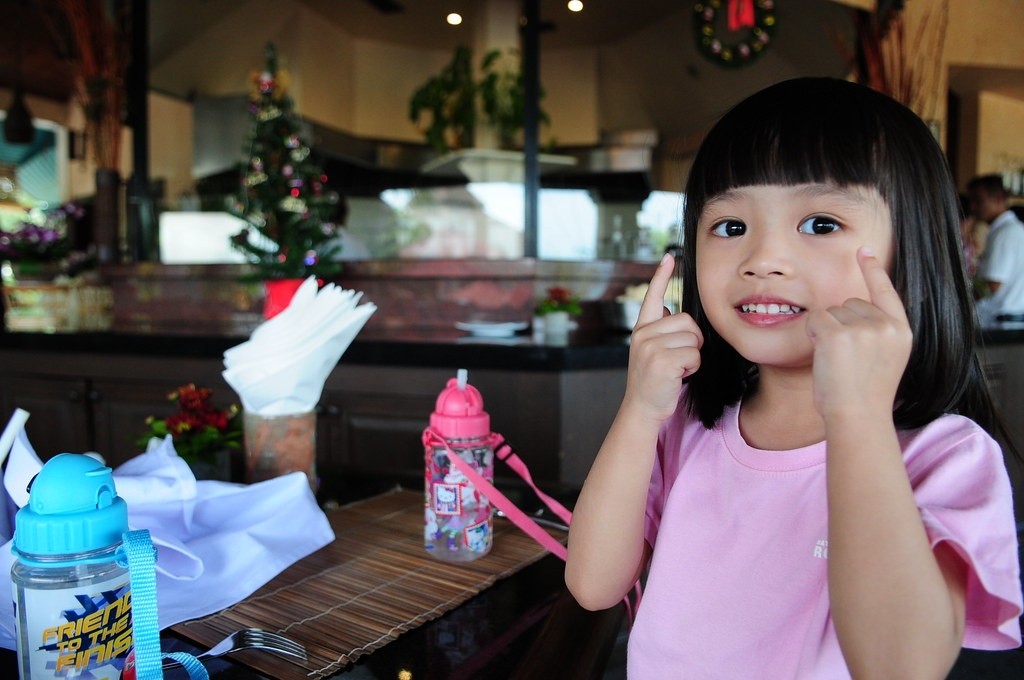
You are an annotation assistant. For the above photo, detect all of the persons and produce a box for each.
[956,191,980,273]
[963,170,1024,346]
[561,74,1023,680]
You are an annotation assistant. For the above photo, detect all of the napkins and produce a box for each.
[222,273,377,416]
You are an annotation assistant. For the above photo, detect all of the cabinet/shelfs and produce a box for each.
[0,370,209,470]
[224,395,503,479]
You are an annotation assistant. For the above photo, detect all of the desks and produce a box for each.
[0,478,626,680]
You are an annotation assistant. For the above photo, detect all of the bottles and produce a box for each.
[9,454,139,680]
[423,370,495,561]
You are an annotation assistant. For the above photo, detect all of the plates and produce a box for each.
[455,322,529,336]
